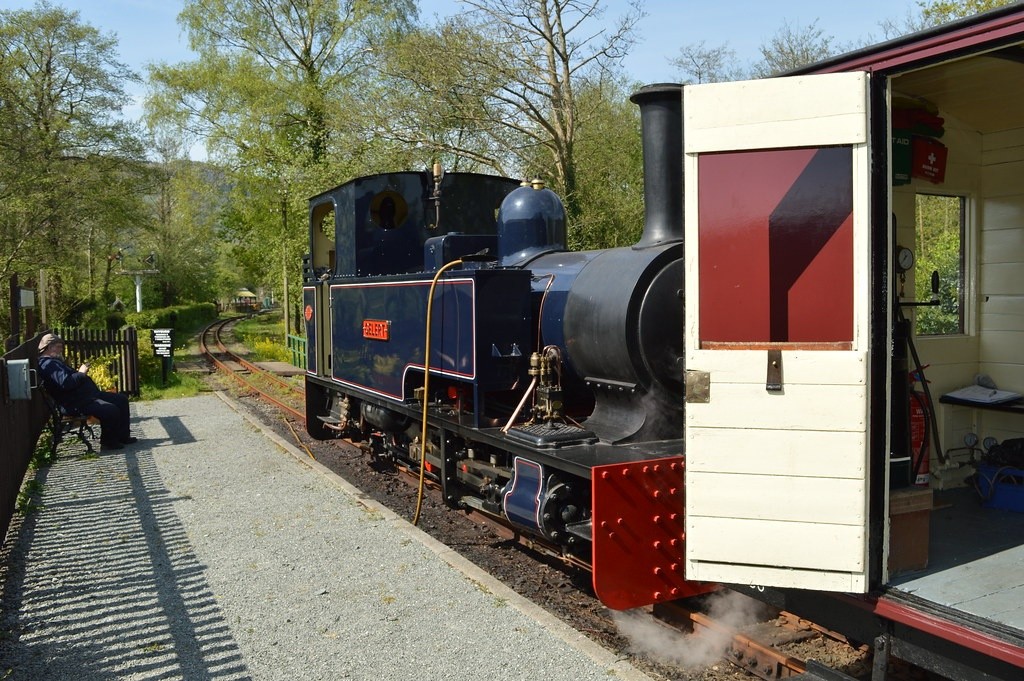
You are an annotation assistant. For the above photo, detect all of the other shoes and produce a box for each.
[120,435,138,444]
[101,444,125,450]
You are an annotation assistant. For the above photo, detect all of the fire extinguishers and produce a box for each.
[909,364,931,487]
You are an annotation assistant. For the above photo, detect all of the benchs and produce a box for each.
[937,385,1024,414]
[42,380,99,453]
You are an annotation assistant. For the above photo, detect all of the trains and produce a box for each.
[302,1,1023,681]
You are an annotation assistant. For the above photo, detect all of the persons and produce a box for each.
[36,333,136,450]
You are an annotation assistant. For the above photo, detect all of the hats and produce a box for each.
[38,333,59,354]
